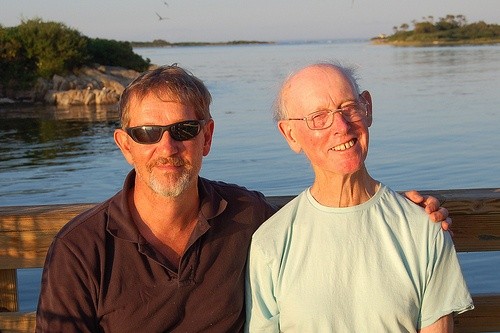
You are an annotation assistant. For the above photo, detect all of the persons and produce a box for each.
[243,60,475,333]
[34,65,453,333]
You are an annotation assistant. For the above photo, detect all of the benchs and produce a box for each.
[2,187,500,332]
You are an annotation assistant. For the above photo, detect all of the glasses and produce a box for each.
[126,116,206,144]
[282,94,370,130]
